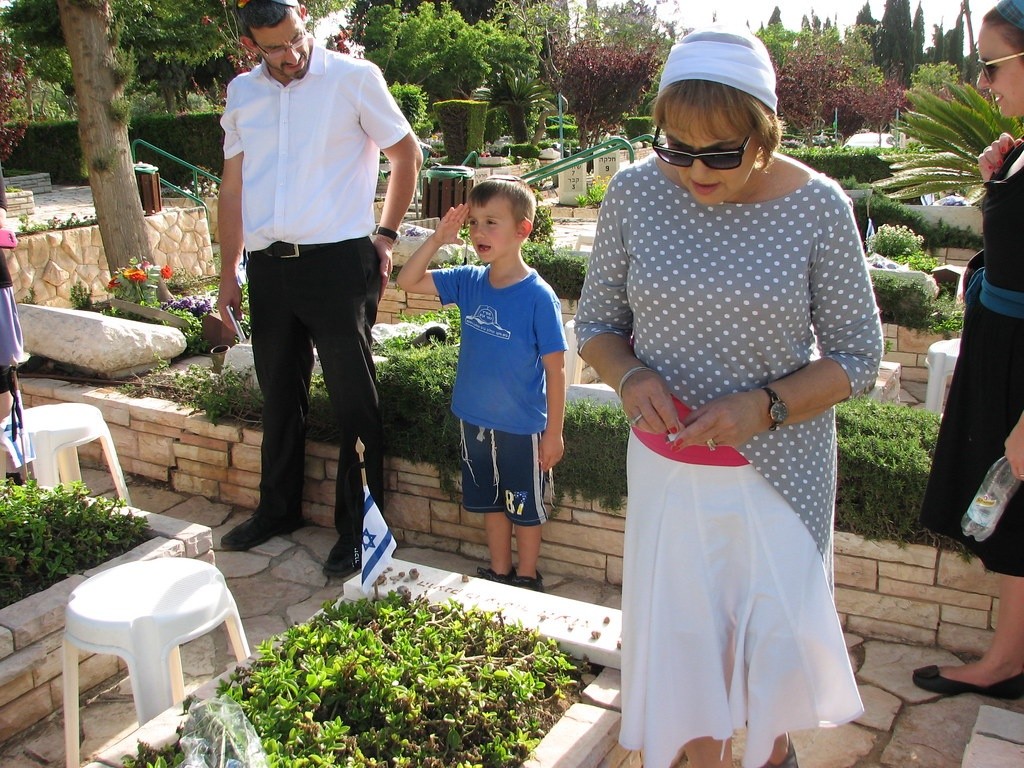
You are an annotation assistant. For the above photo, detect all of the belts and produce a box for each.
[261,242,329,259]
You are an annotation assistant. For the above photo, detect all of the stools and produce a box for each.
[0,401,132,507]
[923,337,961,414]
[61,557,251,768]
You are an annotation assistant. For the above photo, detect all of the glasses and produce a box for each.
[975,50,1024,85]
[254,27,308,53]
[650,121,756,171]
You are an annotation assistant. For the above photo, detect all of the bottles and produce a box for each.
[960,456,1021,543]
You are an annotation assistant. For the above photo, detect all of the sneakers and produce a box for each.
[477,564,545,592]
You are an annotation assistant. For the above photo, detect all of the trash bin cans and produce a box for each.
[132,161,162,216]
[421,166,476,219]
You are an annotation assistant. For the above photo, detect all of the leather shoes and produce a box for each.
[324,535,361,577]
[222,513,308,552]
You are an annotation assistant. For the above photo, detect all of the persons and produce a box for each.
[396,174,569,593]
[573,22,883,768]
[217,0,424,578]
[912,0,1023,701]
[0,164,28,485]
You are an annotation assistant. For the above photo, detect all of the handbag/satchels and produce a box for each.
[963,135,1024,298]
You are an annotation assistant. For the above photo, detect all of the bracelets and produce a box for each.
[376,227,398,241]
[618,366,657,399]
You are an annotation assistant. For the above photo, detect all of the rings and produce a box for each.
[707,438,717,453]
[631,413,643,427]
[1019,474,1024,477]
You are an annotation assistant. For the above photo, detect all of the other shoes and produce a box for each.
[765,734,798,768]
[5,472,24,486]
[912,665,1024,700]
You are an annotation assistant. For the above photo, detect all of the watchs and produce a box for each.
[760,386,790,433]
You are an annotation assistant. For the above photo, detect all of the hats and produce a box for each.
[658,23,778,113]
[235,0,297,18]
